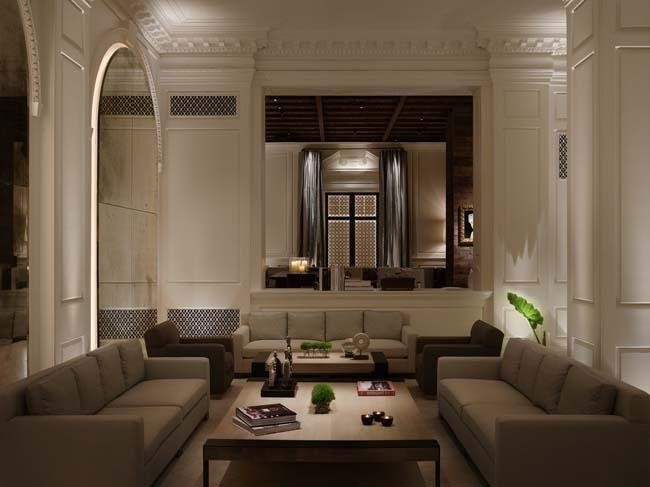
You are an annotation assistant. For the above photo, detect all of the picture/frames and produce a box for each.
[458,206,473,247]
[289,256,310,274]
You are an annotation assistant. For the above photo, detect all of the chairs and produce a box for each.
[415,319,504,400]
[424,267,449,289]
[144,320,235,399]
[380,277,416,287]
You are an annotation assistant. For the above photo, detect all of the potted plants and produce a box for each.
[312,383,335,413]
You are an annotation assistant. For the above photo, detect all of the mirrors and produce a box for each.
[1,0,30,347]
[96,46,159,358]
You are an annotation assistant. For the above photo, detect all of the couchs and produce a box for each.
[1,306,29,346]
[437,337,650,486]
[232,309,419,379]
[0,339,211,486]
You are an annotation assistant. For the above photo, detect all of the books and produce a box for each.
[236,403,296,427]
[357,380,395,396]
[232,416,301,436]
[317,264,375,290]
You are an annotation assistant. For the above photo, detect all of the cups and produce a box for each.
[361,414,373,424]
[381,415,393,426]
[373,411,385,421]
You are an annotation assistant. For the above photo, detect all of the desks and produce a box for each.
[266,268,317,288]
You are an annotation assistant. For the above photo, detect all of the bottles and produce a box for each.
[268,345,295,386]
